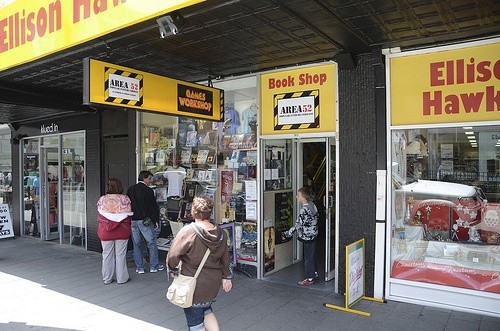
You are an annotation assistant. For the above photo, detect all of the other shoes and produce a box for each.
[315,275,318,280]
[298,279,315,285]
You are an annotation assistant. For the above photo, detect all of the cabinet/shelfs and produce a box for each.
[141,126,259,262]
[45,163,85,225]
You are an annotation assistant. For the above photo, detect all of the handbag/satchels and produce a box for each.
[166,274,197,308]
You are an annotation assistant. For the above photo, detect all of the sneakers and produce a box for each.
[150,264,164,272]
[136,269,144,273]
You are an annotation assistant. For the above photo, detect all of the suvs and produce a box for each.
[391,162,487,232]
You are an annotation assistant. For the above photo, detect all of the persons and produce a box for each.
[282,187,319,286]
[97,178,132,285]
[167,194,233,331]
[126,171,165,274]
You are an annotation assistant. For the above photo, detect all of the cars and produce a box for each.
[150,170,247,220]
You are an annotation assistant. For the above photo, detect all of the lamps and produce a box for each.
[156,13,189,39]
